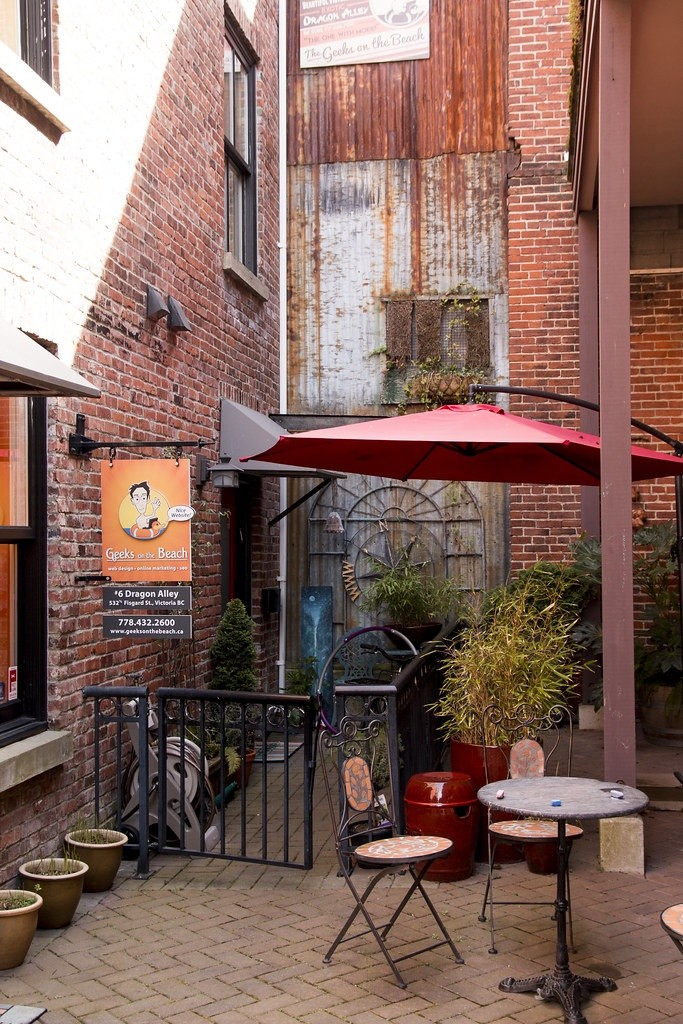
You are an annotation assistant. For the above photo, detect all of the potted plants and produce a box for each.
[18,845,90,928]
[416,280,482,395]
[630,520,683,742]
[206,598,262,788]
[64,818,129,893]
[0,883,43,971]
[426,564,599,866]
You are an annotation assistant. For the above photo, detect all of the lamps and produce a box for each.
[194,453,243,487]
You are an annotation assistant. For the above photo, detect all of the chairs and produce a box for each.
[659,901,682,955]
[320,716,465,990]
[476,705,579,952]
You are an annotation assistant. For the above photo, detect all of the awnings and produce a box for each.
[240,402,683,487]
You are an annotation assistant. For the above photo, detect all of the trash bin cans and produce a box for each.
[403,770,480,884]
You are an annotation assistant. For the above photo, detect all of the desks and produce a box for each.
[475,777,649,1024]
